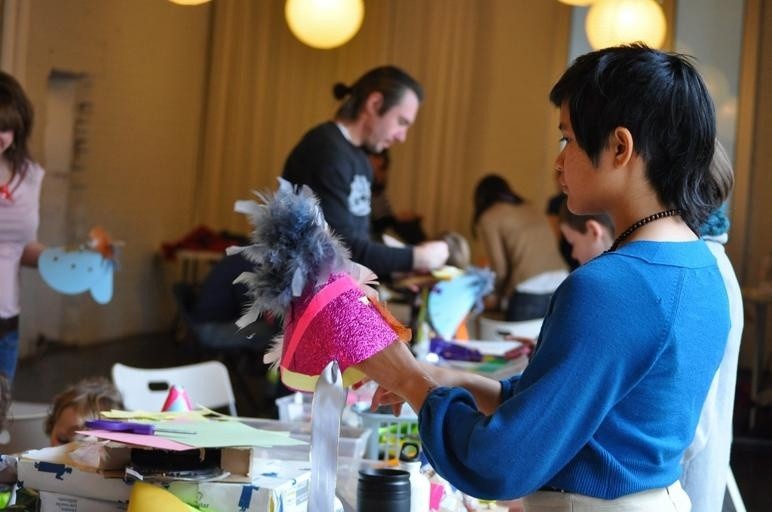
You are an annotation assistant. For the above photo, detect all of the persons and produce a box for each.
[0,69,52,456]
[467,174,570,323]
[190,255,281,411]
[44,378,126,445]
[677,133,747,511]
[546,180,618,273]
[280,64,449,274]
[362,143,432,246]
[348,45,746,511]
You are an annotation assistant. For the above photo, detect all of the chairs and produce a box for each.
[111,360,239,419]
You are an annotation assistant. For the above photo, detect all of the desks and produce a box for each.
[175,246,471,355]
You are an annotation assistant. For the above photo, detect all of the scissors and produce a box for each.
[84,418,199,436]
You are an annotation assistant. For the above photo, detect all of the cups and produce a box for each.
[357,468,411,512]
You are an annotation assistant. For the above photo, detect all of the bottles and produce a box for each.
[401,461,430,511]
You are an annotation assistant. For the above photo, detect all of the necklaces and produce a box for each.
[609,207,684,250]
